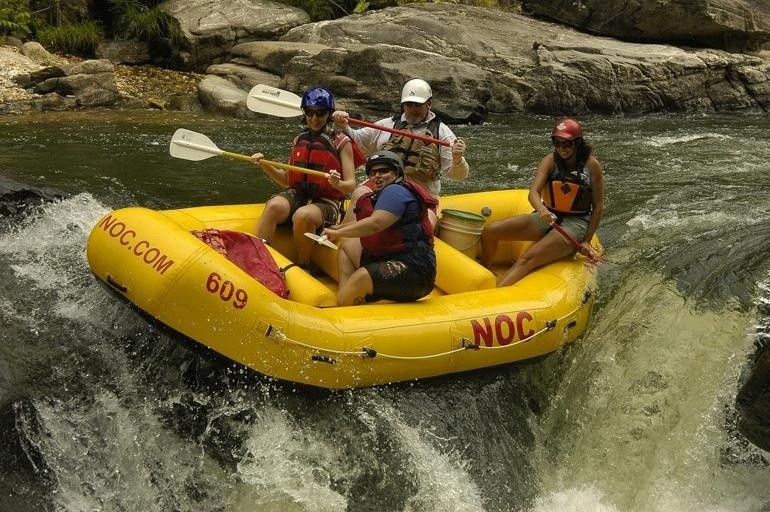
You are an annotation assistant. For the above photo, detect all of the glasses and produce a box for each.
[554,141,573,148]
[304,109,327,117]
[406,102,423,107]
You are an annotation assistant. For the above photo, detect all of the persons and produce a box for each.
[320,149,437,307]
[252,88,356,278]
[477,118,604,288]
[333,78,470,238]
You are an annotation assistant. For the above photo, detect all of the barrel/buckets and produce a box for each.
[439,209,487,259]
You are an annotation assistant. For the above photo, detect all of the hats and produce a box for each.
[400,78,432,103]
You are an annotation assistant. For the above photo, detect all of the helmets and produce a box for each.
[365,149,405,179]
[300,86,335,111]
[549,119,583,141]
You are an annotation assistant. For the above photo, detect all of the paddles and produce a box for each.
[246,84,454,149]
[170,127,330,181]
[305,231,338,250]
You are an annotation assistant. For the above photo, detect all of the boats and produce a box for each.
[86,189,604,390]
